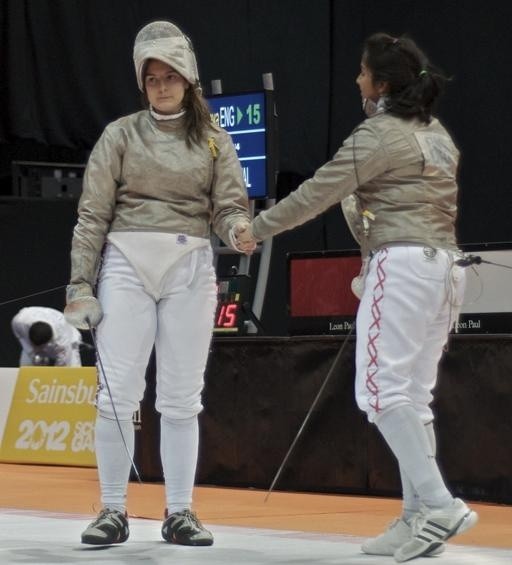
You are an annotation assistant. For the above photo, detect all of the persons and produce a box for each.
[11,305,83,368]
[63,21,257,544]
[238,36,477,564]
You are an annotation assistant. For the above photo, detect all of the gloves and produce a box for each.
[64,282,102,331]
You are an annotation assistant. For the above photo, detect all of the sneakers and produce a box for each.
[393,497,479,563]
[361,517,445,557]
[161,508,213,547]
[81,507,131,545]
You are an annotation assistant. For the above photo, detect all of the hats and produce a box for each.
[133,20,199,91]
